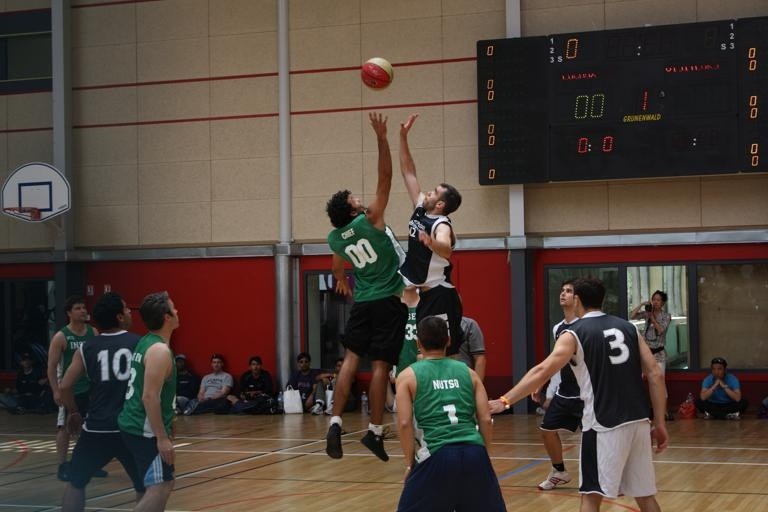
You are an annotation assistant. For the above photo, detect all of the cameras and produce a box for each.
[645,304,652,312]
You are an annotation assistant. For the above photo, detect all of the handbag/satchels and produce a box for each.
[284,386,303,414]
[325,390,334,409]
[679,400,696,419]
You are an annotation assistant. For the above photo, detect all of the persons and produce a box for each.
[396,315,508,512]
[2,350,55,417]
[324,112,410,462]
[488,276,671,511]
[58,291,144,510]
[47,297,110,480]
[532,279,588,492]
[700,358,749,419]
[395,114,466,357]
[173,352,357,416]
[391,284,421,418]
[630,291,671,368]
[117,291,181,511]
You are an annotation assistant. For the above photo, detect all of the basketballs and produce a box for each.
[362,59,393,89]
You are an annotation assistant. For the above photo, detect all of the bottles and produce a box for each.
[277,391,283,412]
[361,390,369,415]
[686,392,693,402]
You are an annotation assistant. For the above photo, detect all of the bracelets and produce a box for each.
[501,394,510,410]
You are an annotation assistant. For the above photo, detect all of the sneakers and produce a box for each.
[94,469,108,478]
[57,462,72,480]
[326,423,343,458]
[311,404,323,414]
[360,430,389,462]
[704,411,713,419]
[727,411,741,421]
[324,406,333,415]
[538,466,572,490]
